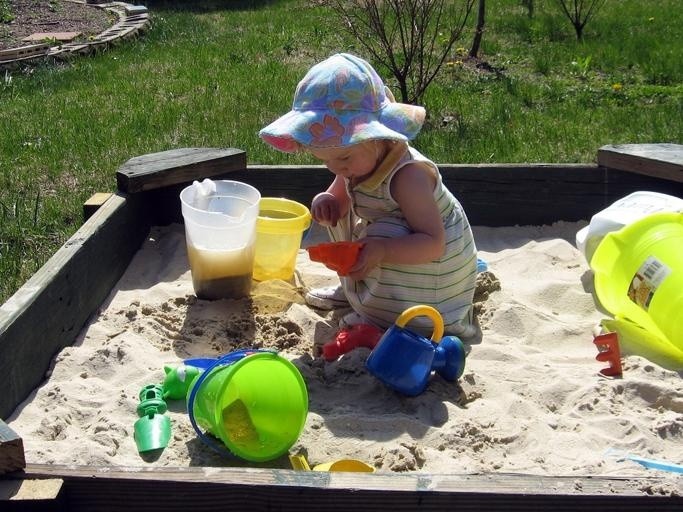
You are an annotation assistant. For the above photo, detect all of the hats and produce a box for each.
[257,53,426,155]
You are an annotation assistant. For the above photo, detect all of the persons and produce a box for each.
[260,54,476,334]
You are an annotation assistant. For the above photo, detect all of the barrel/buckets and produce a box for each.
[589,211,683,354]
[185,350,309,460]
[179,176,261,300]
[254,198,311,284]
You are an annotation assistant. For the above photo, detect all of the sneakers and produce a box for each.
[339,311,368,330]
[306,282,352,309]
